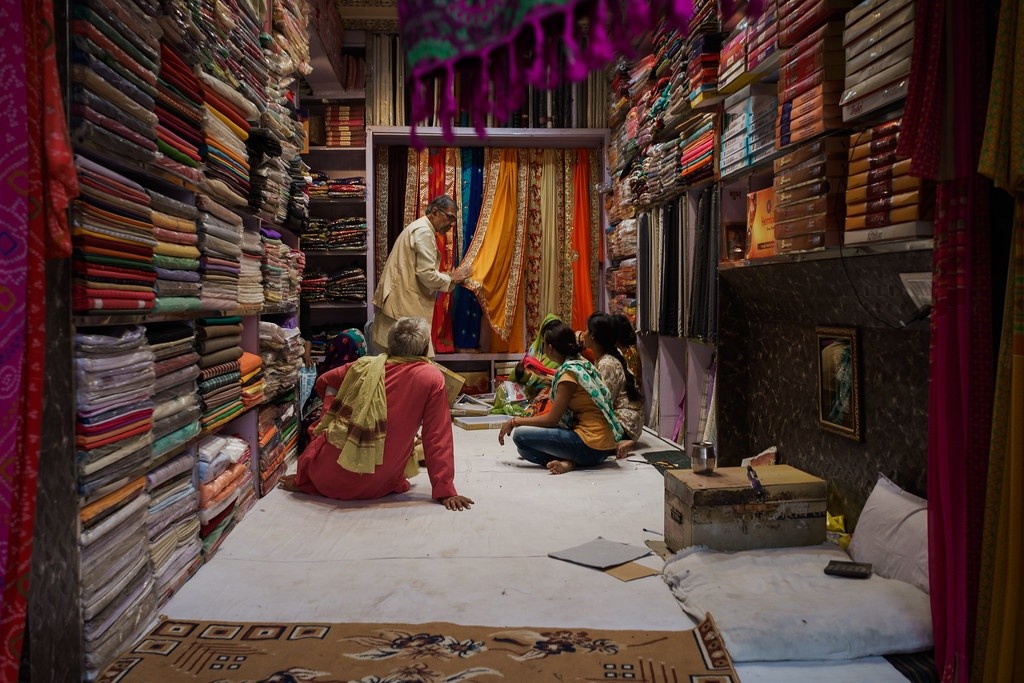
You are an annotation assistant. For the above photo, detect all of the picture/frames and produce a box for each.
[814,326,859,443]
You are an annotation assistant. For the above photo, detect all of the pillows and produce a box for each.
[661,543,932,661]
[845,471,929,596]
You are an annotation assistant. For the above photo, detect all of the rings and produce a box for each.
[450,500,453,503]
[499,435,502,437]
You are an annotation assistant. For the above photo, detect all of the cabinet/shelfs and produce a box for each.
[26,1,376,683]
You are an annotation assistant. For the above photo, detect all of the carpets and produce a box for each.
[89,611,746,683]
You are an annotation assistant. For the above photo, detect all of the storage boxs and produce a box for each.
[718,1,934,260]
[664,464,828,553]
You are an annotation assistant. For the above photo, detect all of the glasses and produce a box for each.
[438,209,457,222]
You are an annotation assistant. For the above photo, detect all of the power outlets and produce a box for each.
[899,272,933,318]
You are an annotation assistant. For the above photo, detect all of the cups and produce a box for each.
[692,442,715,473]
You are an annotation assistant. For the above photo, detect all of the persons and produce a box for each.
[498,311,645,475]
[278,316,474,511]
[299,296,316,368]
[371,195,474,356]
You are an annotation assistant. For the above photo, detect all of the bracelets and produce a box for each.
[509,418,519,428]
